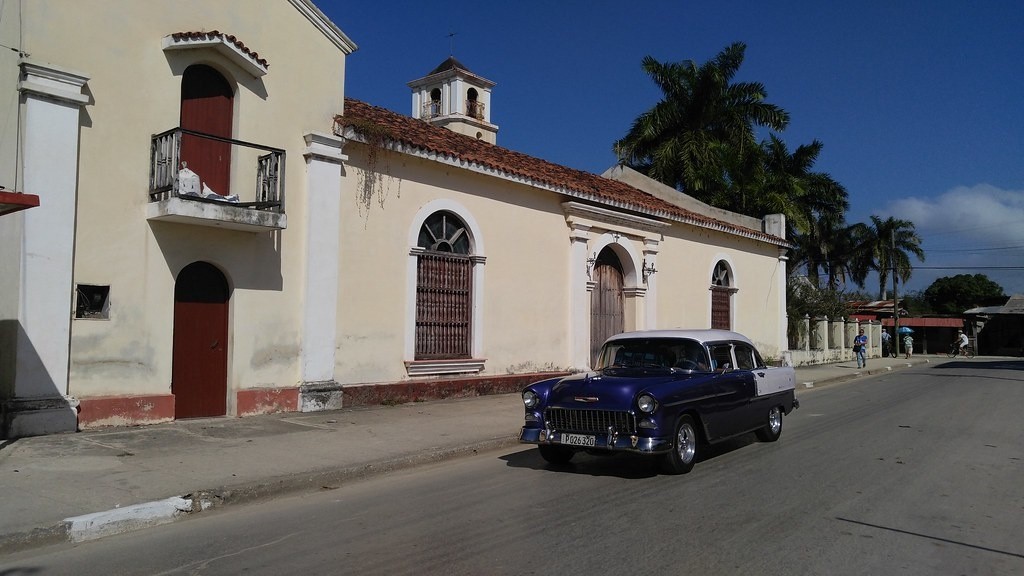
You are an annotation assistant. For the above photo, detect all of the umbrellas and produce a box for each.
[896,327,915,333]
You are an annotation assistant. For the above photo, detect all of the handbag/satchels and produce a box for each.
[882,334,888,342]
[853,345,861,352]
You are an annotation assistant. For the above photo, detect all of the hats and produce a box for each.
[958,330,963,332]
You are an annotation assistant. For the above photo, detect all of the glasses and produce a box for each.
[860,332,864,333]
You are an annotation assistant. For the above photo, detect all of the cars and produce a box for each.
[520,328,802,473]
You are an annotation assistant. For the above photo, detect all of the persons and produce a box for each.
[882,329,891,342]
[903,333,914,359]
[854,329,867,369]
[955,330,969,356]
[674,345,717,372]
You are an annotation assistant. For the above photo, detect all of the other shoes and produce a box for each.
[863,363,866,368]
[959,349,962,351]
[905,358,907,359]
[857,367,861,369]
[965,352,968,355]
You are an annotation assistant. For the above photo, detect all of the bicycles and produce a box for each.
[882,341,897,358]
[947,342,976,359]
